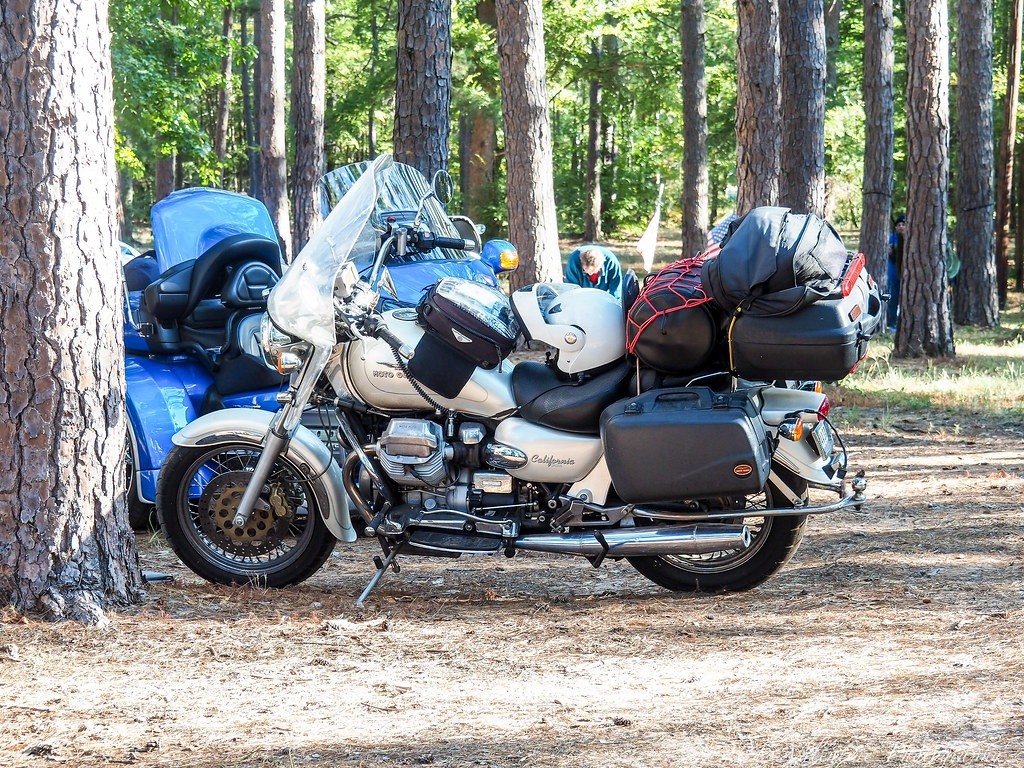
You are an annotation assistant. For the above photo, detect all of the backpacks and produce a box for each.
[414,275,528,371]
[945,240,960,279]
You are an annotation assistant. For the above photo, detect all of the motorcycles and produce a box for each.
[156,152,891,607]
[119,161,519,532]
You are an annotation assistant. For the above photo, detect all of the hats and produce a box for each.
[894,217,905,226]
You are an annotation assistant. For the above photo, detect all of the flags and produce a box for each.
[708,214,737,259]
[636,192,661,274]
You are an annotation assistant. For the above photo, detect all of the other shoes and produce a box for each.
[886,324,897,332]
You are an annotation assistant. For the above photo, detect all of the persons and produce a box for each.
[886,216,907,333]
[565,245,623,303]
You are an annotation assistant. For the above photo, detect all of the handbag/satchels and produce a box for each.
[701,205,847,320]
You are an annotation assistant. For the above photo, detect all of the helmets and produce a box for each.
[510,277,626,381]
[480,239,519,276]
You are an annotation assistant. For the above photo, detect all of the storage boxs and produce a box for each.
[602,386,776,507]
[730,264,889,389]
